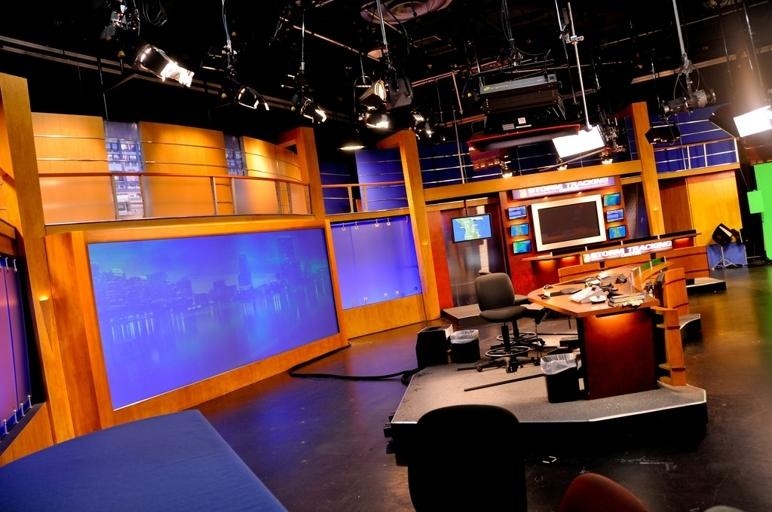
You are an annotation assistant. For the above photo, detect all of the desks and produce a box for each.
[521,229,711,284]
[526,256,690,400]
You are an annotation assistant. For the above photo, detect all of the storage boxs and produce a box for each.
[417,324,453,369]
[450,329,481,363]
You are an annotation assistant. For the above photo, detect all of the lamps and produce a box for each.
[545,0,627,171]
[217,0,269,111]
[452,73,472,141]
[645,55,681,144]
[280,0,328,123]
[733,0,772,138]
[662,0,717,113]
[502,155,513,177]
[118,0,195,89]
[338,0,446,149]
[708,0,740,139]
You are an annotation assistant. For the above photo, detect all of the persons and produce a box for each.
[559,472,649,512]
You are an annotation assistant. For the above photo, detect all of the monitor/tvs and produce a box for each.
[606,208,624,223]
[451,213,493,244]
[603,192,621,208]
[530,194,607,252]
[608,224,626,241]
[507,205,527,221]
[510,223,529,238]
[512,239,532,255]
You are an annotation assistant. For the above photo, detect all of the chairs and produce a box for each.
[474,270,545,373]
[408,405,527,512]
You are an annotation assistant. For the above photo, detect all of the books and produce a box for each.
[608,291,646,307]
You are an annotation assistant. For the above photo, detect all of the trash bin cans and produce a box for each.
[541,353,579,403]
[450,329,480,370]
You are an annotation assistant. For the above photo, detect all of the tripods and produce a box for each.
[712,246,738,270]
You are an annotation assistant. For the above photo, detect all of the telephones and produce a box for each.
[570,285,602,304]
